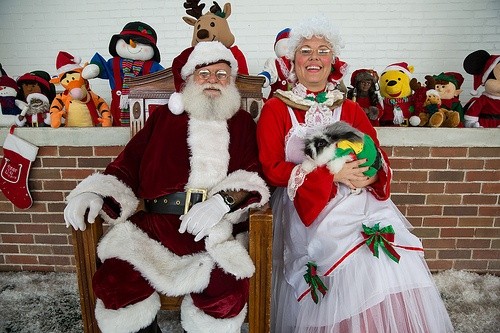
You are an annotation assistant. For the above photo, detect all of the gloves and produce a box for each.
[63,192,104,231]
[178,194,230,242]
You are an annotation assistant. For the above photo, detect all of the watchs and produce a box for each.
[220,193,235,207]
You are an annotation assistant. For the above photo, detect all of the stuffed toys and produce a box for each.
[81,21,166,127]
[377,62,464,128]
[258,28,298,101]
[49,51,113,128]
[182,0,248,75]
[0,63,56,127]
[463,50,500,128]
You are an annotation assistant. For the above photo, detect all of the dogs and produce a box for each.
[303,121,383,176]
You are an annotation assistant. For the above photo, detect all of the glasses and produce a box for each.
[189,69,233,81]
[296,45,333,56]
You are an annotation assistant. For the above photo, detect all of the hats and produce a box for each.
[167,41,238,115]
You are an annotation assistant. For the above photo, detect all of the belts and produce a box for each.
[135,189,208,215]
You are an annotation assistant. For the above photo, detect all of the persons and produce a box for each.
[64,41,271,333]
[257,22,454,333]
[347,69,384,127]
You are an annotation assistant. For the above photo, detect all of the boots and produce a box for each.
[134,314,162,333]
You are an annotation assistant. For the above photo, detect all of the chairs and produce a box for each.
[72,66,273,333]
